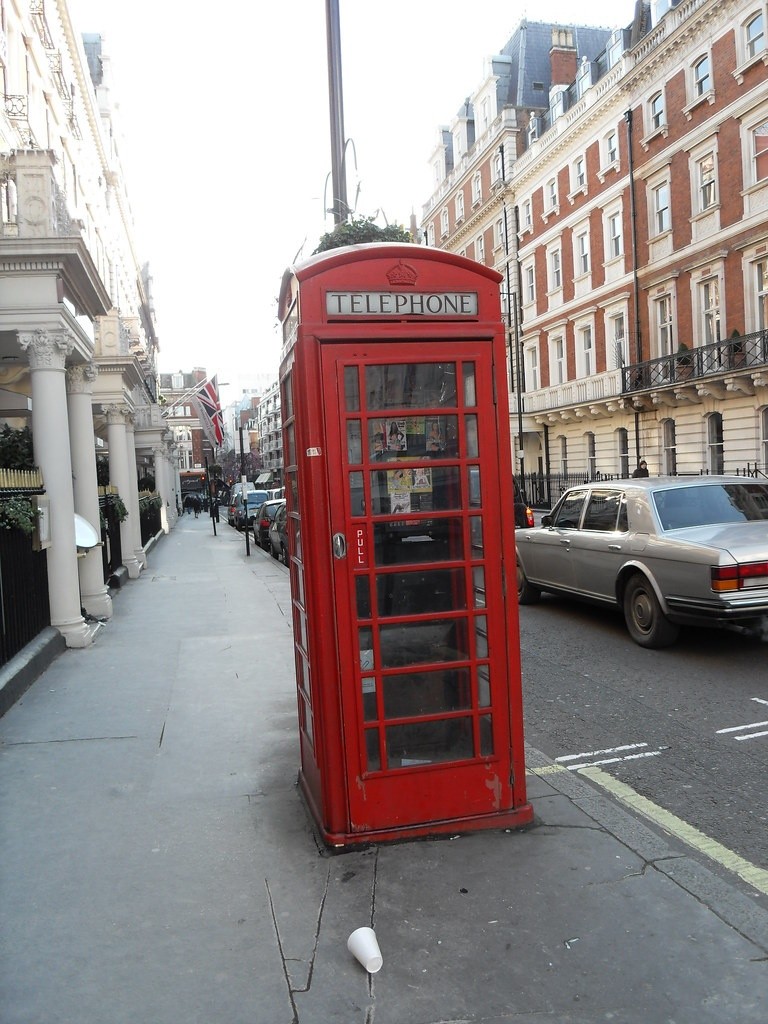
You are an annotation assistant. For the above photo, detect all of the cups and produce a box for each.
[347,927,383,973]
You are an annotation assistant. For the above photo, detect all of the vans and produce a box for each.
[230,482,255,506]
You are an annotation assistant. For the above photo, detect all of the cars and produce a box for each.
[515,475,768,651]
[249,498,287,551]
[224,494,237,526]
[425,470,535,545]
[264,502,287,567]
[232,490,269,531]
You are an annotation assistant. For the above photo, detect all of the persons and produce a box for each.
[632,461,649,478]
[388,422,405,450]
[184,494,203,518]
[429,423,444,451]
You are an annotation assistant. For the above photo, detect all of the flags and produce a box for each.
[189,374,224,449]
